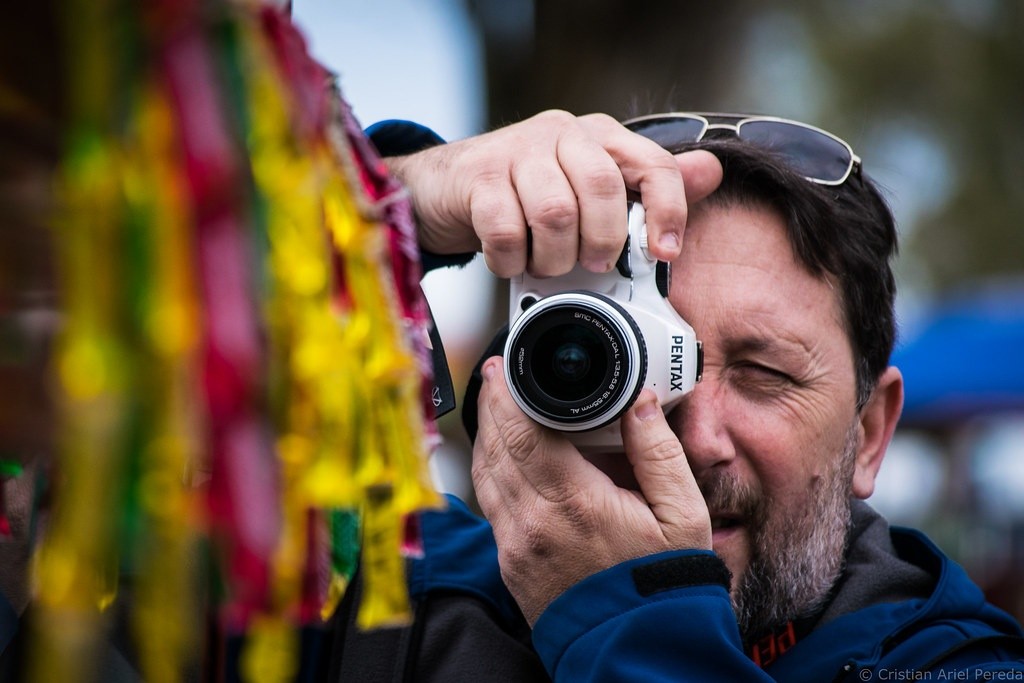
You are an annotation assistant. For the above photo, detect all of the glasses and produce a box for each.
[585,109,866,200]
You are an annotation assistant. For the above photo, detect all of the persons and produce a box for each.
[24,110,1024,683]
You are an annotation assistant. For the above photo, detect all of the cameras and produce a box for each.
[502,189,704,452]
